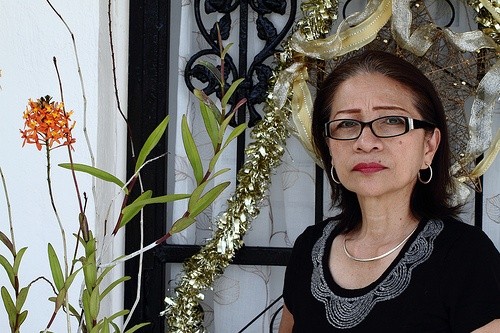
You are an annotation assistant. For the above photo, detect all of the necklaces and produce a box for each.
[341,222,419,263]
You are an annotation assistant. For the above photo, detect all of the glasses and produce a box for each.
[322,114,435,140]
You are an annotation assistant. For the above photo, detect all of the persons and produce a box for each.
[276,48,500,332]
